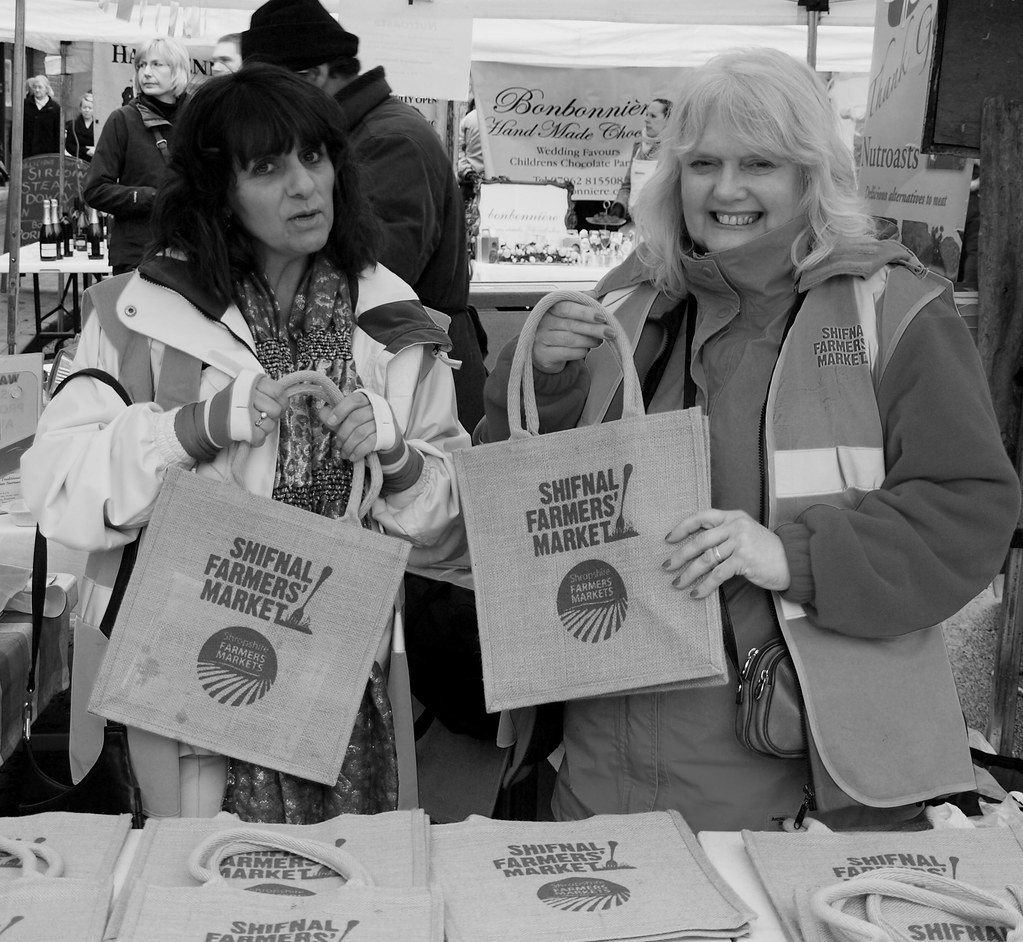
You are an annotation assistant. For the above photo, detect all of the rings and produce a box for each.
[713,546,723,563]
[255,412,267,426]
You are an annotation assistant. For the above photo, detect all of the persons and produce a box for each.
[457,109,484,206]
[221,0,488,446]
[9,74,60,159]
[84,32,195,276]
[610,98,674,223]
[472,46,1021,831]
[210,32,242,76]
[65,93,94,162]
[20,59,480,822]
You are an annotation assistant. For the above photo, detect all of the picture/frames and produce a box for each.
[468,174,576,261]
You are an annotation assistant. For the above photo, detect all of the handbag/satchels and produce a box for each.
[87,370,413,787]
[0,800,1023,942]
[452,290,729,713]
[737,638,812,758]
[0,687,143,829]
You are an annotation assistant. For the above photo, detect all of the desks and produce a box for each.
[468,259,609,310]
[0,611,70,768]
[697,831,789,942]
[0,239,112,358]
[0,809,758,942]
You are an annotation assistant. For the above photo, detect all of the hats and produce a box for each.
[240,0,359,73]
[28,74,54,98]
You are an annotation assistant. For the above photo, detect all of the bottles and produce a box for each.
[59,212,74,257]
[50,199,64,259]
[71,197,80,248]
[40,200,57,260]
[107,213,114,248]
[86,207,104,259]
[75,202,88,251]
